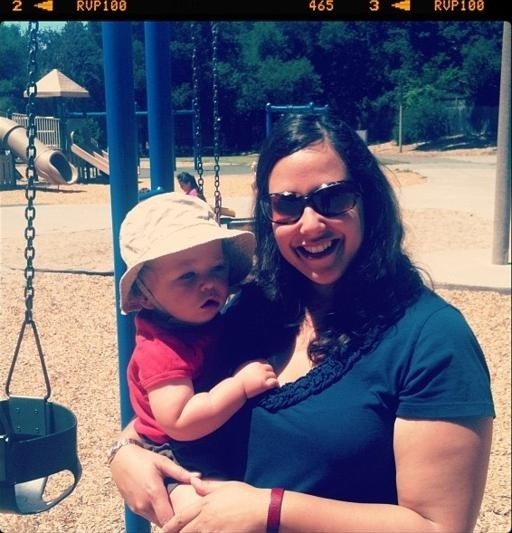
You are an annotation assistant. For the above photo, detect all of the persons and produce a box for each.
[117,191,279,517]
[105,107,496,533]
[177,171,202,197]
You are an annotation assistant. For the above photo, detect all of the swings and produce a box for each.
[189,20,221,226]
[0,20,82,514]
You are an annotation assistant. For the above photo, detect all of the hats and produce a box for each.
[119,193,256,316]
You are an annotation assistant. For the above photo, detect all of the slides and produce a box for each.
[71,144,109,176]
[0,116,79,185]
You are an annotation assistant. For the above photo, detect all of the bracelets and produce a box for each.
[265,487,285,533]
[104,438,144,468]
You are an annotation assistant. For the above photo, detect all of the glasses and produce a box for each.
[260,180,360,224]
